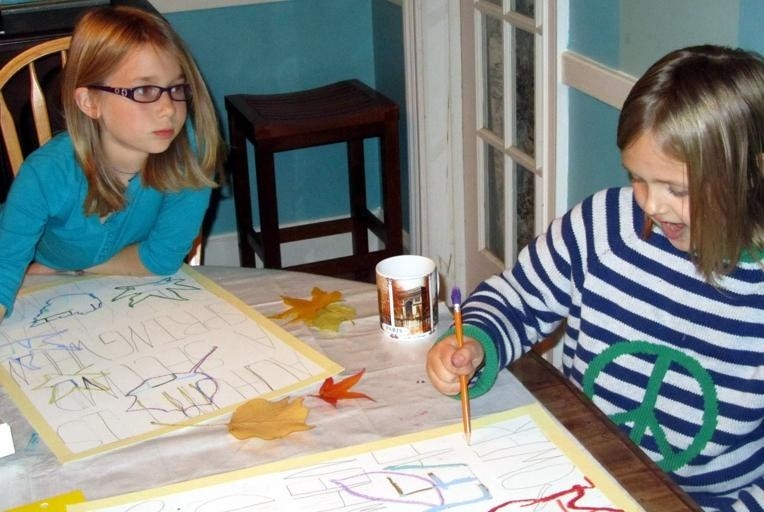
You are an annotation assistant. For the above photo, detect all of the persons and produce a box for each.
[0,6,222,331]
[425,45,764,512]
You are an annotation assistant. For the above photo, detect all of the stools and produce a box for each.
[225,80,402,280]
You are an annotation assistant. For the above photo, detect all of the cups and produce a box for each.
[376,253,441,340]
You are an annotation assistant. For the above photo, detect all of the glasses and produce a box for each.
[87,84,193,103]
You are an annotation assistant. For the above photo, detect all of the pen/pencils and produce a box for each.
[451,289,472,447]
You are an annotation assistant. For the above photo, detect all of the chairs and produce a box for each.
[0,36,202,266]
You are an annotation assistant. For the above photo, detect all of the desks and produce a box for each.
[0,266,703,512]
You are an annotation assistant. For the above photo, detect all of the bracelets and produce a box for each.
[72,269,85,276]
